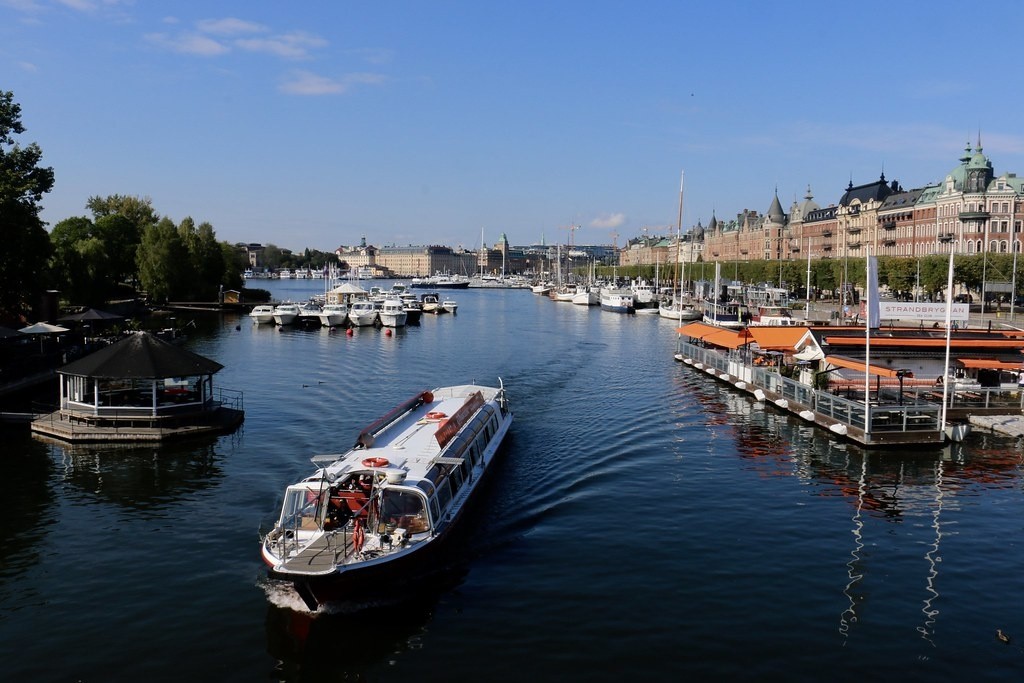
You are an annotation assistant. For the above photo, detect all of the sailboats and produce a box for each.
[466,168,815,331]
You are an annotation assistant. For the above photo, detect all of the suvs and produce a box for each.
[956,294,973,303]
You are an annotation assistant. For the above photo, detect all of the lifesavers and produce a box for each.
[353,520,364,552]
[362,457,389,467]
[426,412,446,418]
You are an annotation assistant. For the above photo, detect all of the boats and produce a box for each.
[442,300,458,313]
[377,299,408,327]
[249,305,275,325]
[298,298,322,327]
[935,418,973,444]
[421,292,440,314]
[403,306,422,325]
[243,265,374,280]
[319,303,348,327]
[369,283,421,309]
[272,303,300,326]
[259,384,514,580]
[347,302,379,328]
[410,264,471,289]
